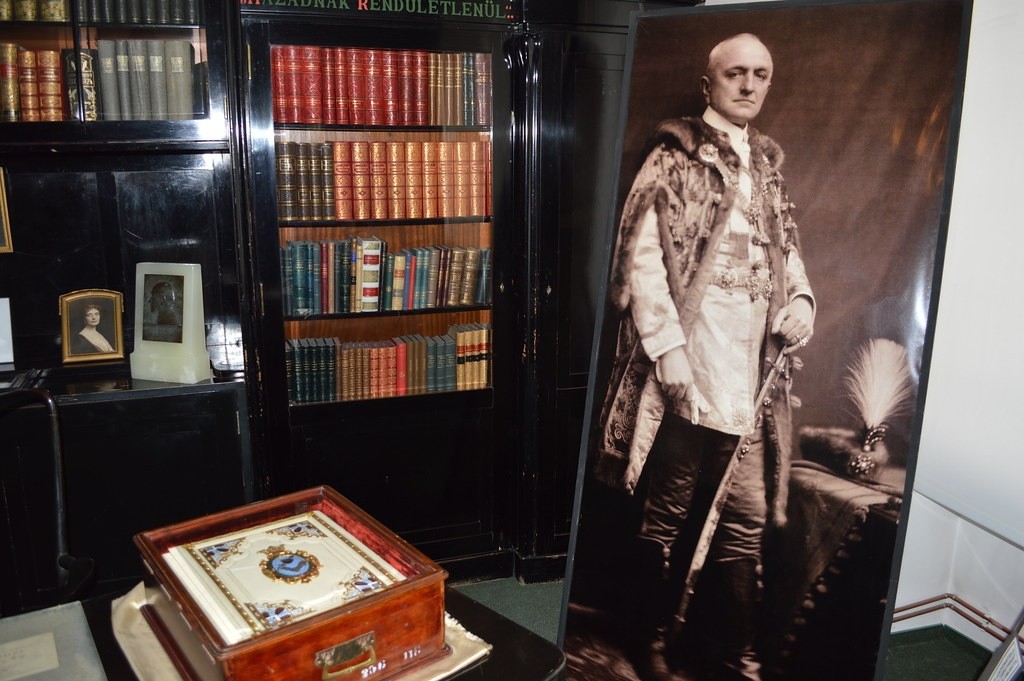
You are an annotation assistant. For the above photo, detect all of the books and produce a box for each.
[0,7,493,407]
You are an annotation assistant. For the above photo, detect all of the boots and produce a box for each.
[627,534,676,681]
[717,556,762,681]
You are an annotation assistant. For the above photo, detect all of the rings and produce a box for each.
[800,338,808,346]
[795,334,802,341]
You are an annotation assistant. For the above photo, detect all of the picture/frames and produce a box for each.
[0,167,13,253]
[557,0,975,681]
[59,289,124,368]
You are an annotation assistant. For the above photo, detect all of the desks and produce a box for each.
[81,584,568,681]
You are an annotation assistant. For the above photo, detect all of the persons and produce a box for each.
[72,306,113,355]
[592,33,817,681]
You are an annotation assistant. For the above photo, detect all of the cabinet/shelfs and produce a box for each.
[0,2,704,611]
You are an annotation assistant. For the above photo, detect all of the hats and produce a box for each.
[801,339,914,485]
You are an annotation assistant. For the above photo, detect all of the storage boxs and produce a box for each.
[134,485,453,681]
